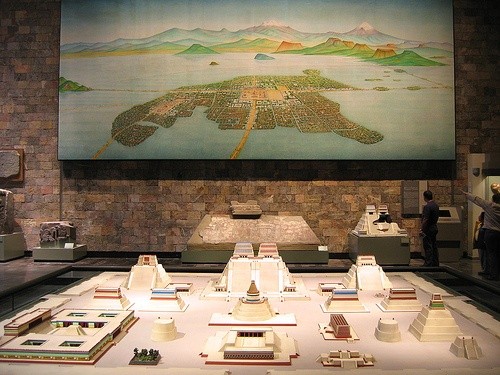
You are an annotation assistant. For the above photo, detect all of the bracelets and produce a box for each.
[420,229,422,230]
[463,192,466,196]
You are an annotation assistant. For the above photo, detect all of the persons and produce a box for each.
[456,183,500,280]
[419,190,440,267]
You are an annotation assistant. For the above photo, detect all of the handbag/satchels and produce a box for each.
[472,228,487,249]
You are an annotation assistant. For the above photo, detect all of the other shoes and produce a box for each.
[487,274,497,279]
[422,264,432,267]
[478,271,488,275]
